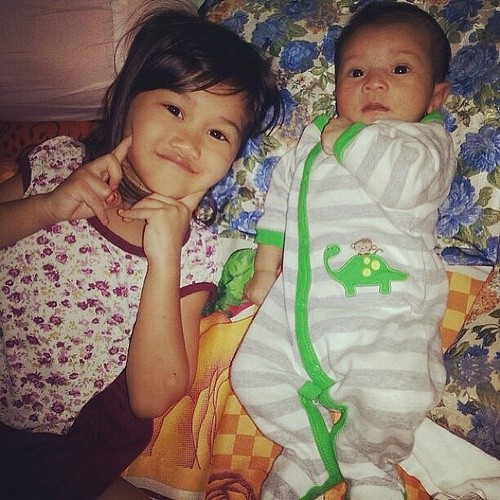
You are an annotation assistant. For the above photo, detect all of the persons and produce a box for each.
[225,0,458,500]
[0,2,276,500]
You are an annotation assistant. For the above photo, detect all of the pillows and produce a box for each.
[0,0,206,125]
[424,260,500,464]
[189,0,500,269]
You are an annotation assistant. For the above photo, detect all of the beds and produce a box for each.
[0,120,500,500]
